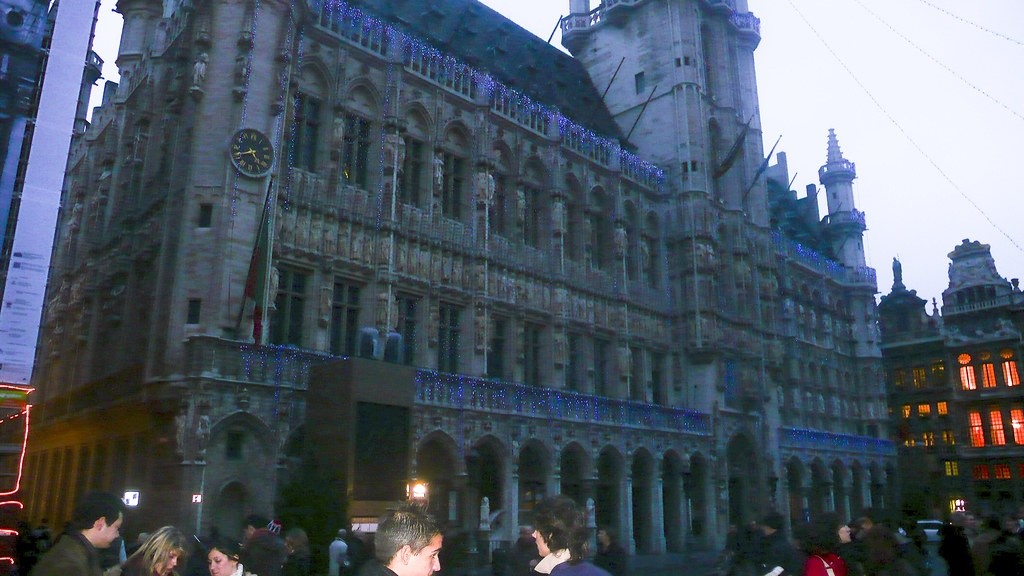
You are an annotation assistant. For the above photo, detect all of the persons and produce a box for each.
[722,508,1024,576]
[13,491,628,576]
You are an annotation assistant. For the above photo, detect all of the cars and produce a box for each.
[899,520,946,543]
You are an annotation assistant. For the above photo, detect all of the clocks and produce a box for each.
[229,128,276,177]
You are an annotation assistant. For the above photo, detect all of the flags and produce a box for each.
[244,179,279,346]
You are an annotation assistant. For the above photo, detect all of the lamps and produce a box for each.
[483,416,492,429]
[279,393,293,418]
[432,410,443,425]
[235,386,254,409]
[402,472,429,503]
